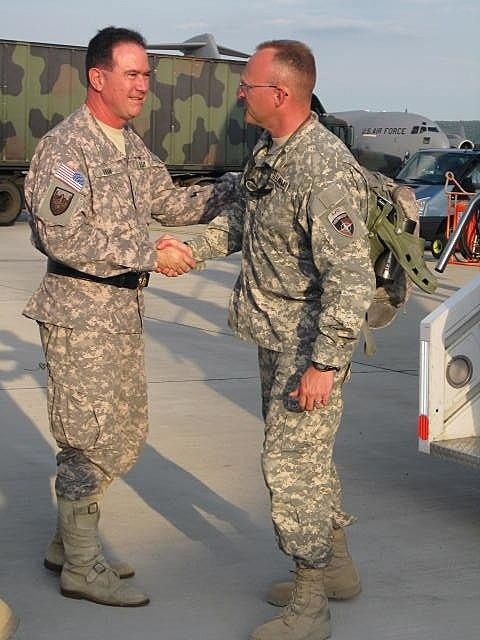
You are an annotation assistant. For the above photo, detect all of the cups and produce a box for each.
[374,216,417,283]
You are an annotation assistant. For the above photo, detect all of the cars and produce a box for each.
[391,147,480,257]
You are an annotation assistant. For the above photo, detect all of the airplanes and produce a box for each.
[149,33,474,177]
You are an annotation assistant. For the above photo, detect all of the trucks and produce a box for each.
[0,39,355,226]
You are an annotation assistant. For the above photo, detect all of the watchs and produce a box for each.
[314,360,343,371]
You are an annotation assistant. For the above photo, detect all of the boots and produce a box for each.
[268,527,361,606]
[44,495,134,578]
[250,568,332,639]
[56,494,151,607]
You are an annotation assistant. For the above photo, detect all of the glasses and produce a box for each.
[236,82,288,97]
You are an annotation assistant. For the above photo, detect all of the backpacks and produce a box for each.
[360,165,439,356]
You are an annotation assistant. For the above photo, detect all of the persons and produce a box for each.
[20,26,254,608]
[160,41,379,640]
